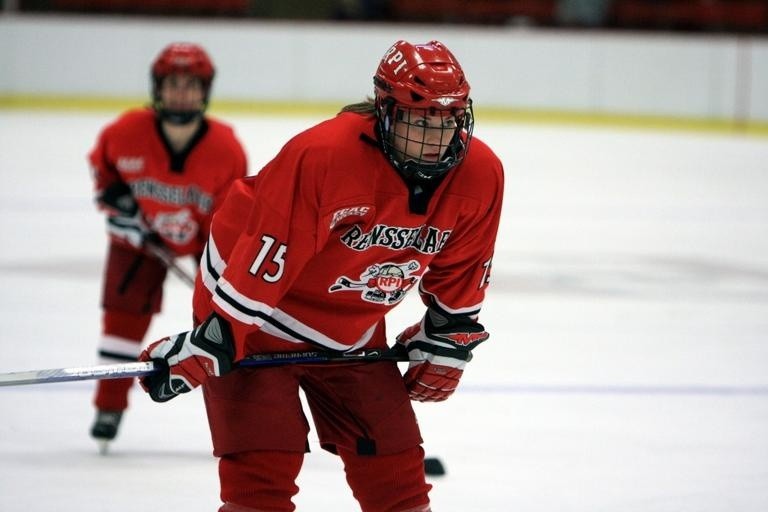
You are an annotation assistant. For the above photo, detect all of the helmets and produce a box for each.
[372,40,476,181]
[149,42,218,125]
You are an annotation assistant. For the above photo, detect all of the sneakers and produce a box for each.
[91,406,124,441]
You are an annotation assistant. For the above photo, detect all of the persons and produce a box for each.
[137,37,505,511]
[84,41,248,441]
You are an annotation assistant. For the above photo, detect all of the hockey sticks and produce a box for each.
[143,238,444,474]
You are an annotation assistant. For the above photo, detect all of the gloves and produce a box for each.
[103,209,154,252]
[388,301,490,404]
[137,311,236,403]
